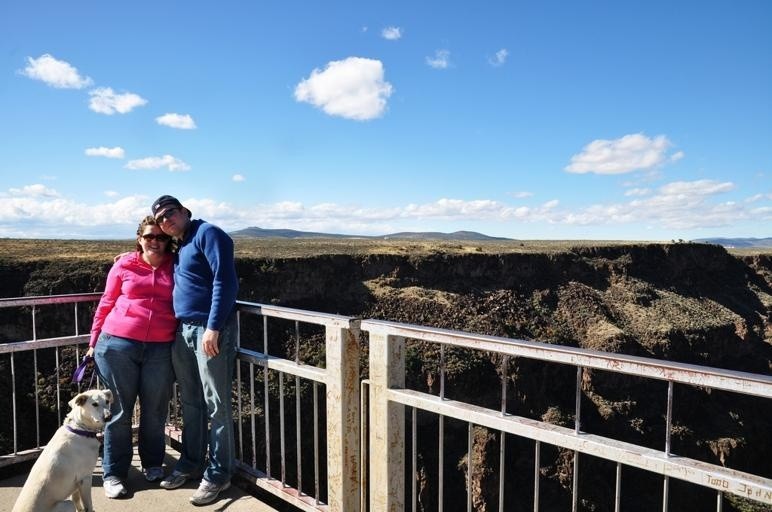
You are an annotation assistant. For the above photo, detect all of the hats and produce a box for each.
[152,195,191,218]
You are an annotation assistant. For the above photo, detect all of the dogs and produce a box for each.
[11,388,114,512]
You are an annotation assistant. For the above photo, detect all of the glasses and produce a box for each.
[142,234,169,242]
[156,208,177,222]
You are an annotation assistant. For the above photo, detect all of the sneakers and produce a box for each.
[103,477,128,498]
[144,466,164,481]
[160,471,190,489]
[191,478,231,505]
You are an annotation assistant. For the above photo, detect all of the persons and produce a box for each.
[84,216,182,500]
[113,194,239,507]
